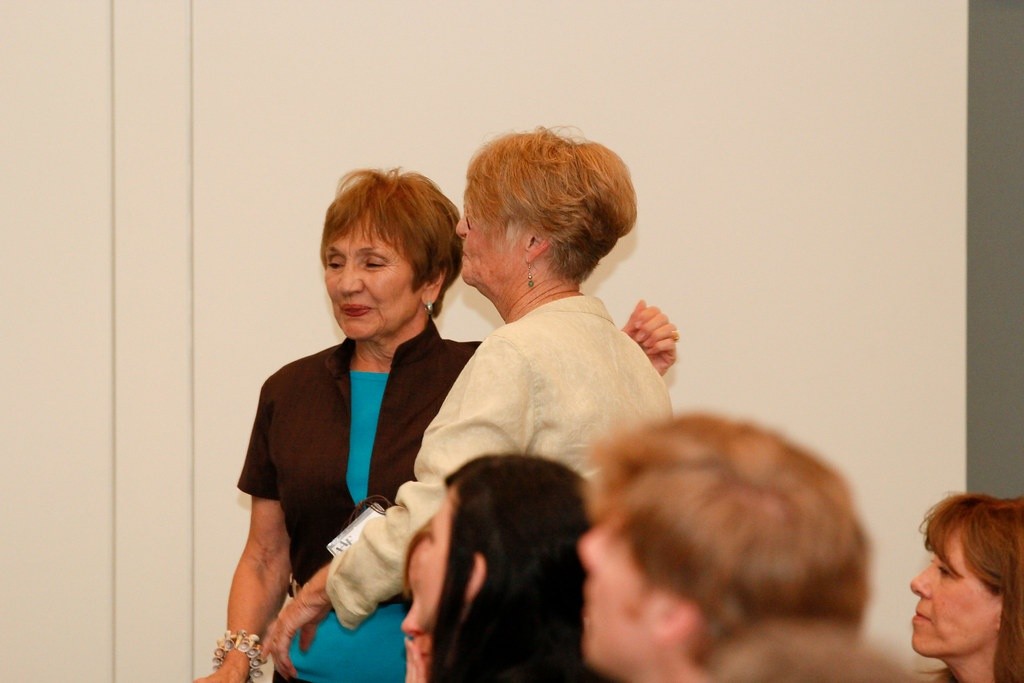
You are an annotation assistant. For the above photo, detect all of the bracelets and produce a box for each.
[212,627,266,683]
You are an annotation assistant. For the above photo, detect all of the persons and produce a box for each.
[260,129,675,678]
[573,415,871,683]
[908,494,1024,683]
[411,455,600,682]
[191,165,681,683]
[402,516,475,683]
